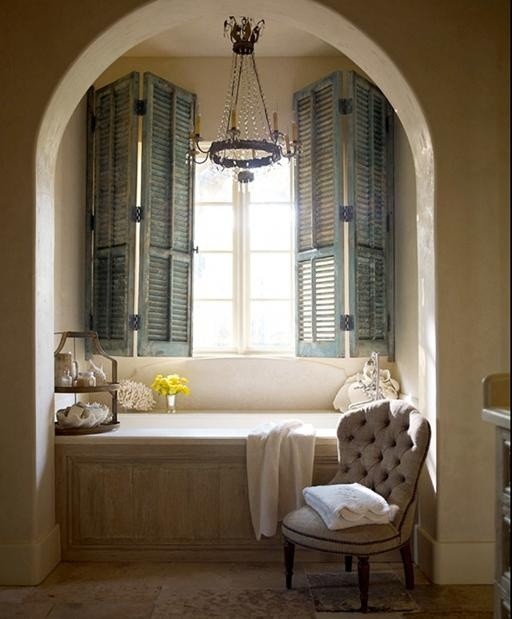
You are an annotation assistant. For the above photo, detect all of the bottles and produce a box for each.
[78,370,96,387]
[61,368,72,387]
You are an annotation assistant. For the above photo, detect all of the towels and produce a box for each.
[247,419,316,539]
[303,482,391,531]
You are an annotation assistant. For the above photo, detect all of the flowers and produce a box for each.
[152,374,190,397]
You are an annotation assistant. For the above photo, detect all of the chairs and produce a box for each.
[281,398,431,610]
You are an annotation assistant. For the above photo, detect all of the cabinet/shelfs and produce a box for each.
[479,372,510,618]
[54,331,120,435]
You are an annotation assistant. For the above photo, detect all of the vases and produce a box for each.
[166,396,177,413]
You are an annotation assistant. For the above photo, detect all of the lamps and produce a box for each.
[186,15,303,194]
